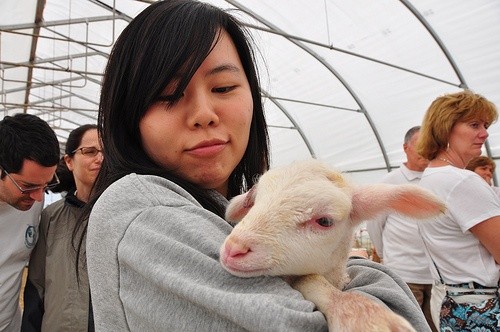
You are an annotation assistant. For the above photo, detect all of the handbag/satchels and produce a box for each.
[439,295,500,332]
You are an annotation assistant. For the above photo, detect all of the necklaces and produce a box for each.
[437,154,462,169]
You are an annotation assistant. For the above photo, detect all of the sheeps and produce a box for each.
[219,157,448,332]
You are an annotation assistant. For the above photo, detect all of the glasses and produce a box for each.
[4,170,60,194]
[71,147,103,157]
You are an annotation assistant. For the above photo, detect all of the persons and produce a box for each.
[0,112,60,332]
[71,0,434,332]
[414,90,499,332]
[17,124,110,332]
[465,155,500,200]
[364,125,442,332]
